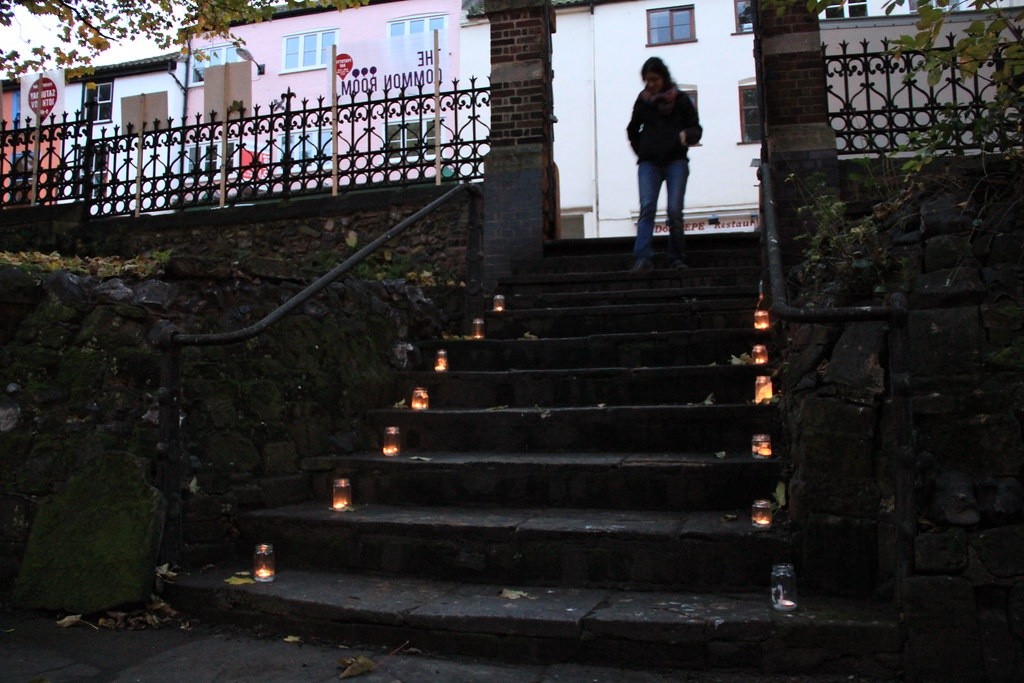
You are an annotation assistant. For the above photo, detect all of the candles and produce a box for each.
[434,349,448,371]
[754,375,773,405]
[770,565,796,612]
[750,500,772,527]
[251,543,274,584]
[411,387,429,410]
[754,310,769,328]
[752,434,772,458]
[384,426,400,456]
[472,319,484,338]
[332,479,352,509]
[493,295,505,311]
[752,344,768,365]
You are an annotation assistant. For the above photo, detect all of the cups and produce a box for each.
[753,345,768,364]
[753,310,769,330]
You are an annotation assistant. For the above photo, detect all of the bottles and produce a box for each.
[752,499,771,527]
[383,427,399,456]
[435,349,449,371]
[473,318,485,338]
[751,434,773,459]
[493,293,504,310]
[252,543,275,582]
[755,376,772,405]
[333,480,352,512]
[411,387,429,410]
[772,564,795,613]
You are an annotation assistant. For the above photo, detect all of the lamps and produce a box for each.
[236,47,265,76]
[708,215,721,228]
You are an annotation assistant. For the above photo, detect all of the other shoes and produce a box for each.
[670,259,689,268]
[629,262,653,277]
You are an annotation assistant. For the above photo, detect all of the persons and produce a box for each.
[627,57,702,271]
